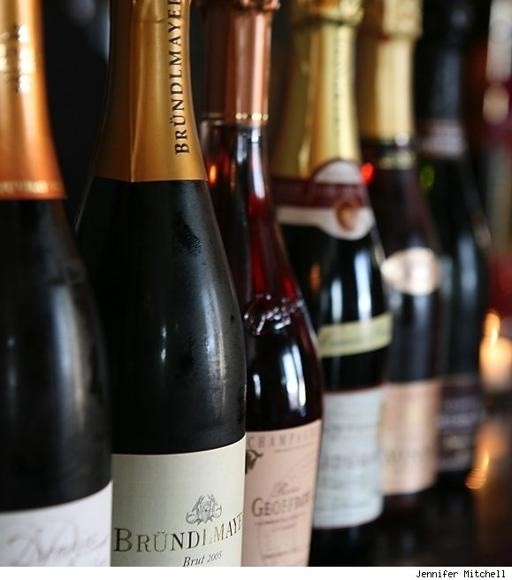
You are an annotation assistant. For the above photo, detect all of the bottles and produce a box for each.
[1,2,117,562]
[193,0,327,563]
[257,1,396,560]
[69,1,248,567]
[344,1,447,527]
[408,1,495,494]
